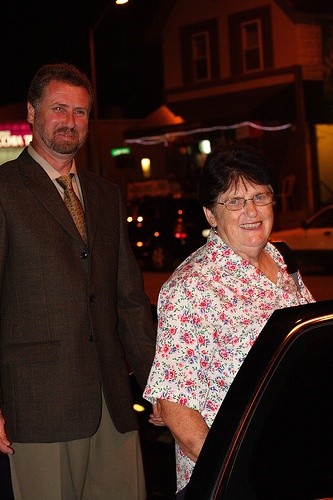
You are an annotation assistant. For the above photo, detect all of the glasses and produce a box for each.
[214,192,274,212]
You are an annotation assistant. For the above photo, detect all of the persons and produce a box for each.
[0,62,169,500]
[143,151,318,491]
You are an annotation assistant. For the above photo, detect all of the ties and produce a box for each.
[55,172,89,244]
